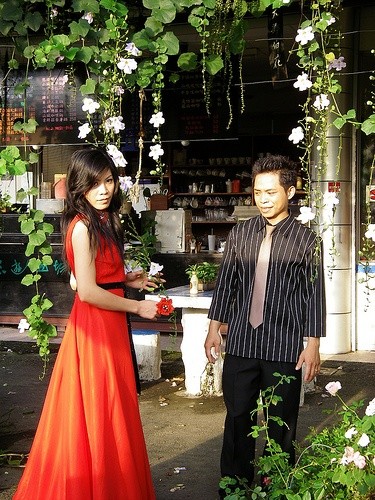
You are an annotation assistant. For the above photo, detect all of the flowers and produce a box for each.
[123,212,175,316]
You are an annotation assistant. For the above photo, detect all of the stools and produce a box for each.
[130,329,162,381]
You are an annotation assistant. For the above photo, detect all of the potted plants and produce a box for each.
[185,261,221,292]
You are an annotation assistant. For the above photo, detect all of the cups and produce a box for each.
[172,167,226,177]
[209,157,252,166]
[173,196,198,208]
[207,235,216,251]
[205,197,228,206]
[229,196,252,206]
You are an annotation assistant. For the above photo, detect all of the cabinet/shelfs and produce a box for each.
[120,136,306,252]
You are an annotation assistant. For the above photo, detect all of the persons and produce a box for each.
[203,155,326,500]
[13,149,162,500]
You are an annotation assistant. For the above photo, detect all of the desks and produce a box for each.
[145,285,225,398]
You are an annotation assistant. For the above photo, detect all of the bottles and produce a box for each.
[205,208,228,220]
[189,271,198,295]
[226,180,240,193]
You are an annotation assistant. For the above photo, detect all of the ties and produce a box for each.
[249,224,275,330]
